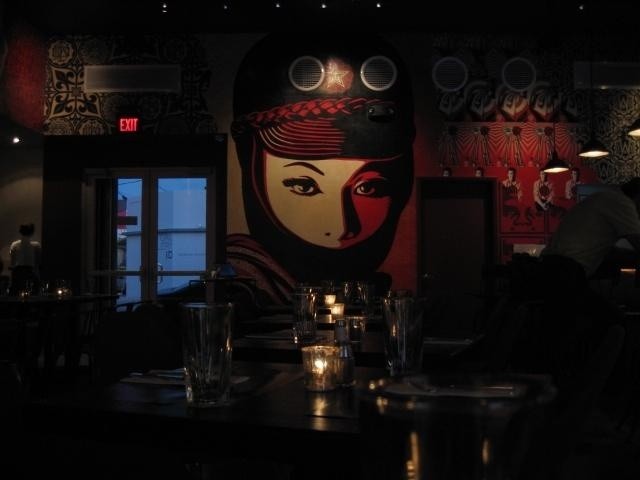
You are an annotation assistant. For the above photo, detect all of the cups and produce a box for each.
[293,292,317,345]
[16,279,71,301]
[299,280,375,320]
[381,296,426,376]
[301,344,339,393]
[181,299,236,410]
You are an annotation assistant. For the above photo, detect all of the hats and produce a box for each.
[232,32,416,161]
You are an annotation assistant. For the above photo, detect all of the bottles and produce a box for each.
[341,346,357,390]
[335,316,366,342]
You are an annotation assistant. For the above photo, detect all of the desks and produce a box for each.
[97,294,528,480]
[0,292,119,383]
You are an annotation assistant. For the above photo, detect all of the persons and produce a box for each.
[442,167,452,177]
[502,167,523,202]
[228,27,415,304]
[564,167,585,201]
[474,166,485,177]
[9,222,43,296]
[534,168,557,212]
[537,176,640,300]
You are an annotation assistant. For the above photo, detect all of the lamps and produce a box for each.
[542,73,640,174]
